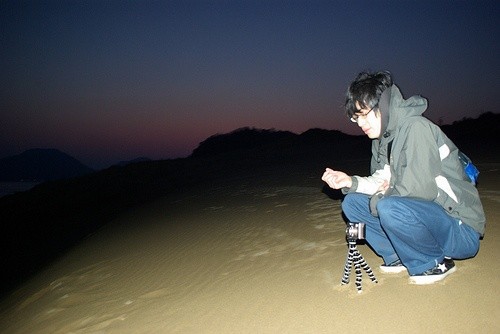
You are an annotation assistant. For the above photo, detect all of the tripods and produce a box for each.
[341,241,378,294]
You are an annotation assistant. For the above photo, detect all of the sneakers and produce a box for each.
[379,260,407,275]
[408,258,456,284]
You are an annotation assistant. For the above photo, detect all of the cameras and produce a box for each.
[345,221,366,240]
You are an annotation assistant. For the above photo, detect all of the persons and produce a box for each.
[321,68,486,285]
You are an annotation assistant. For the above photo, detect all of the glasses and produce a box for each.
[350,104,376,123]
